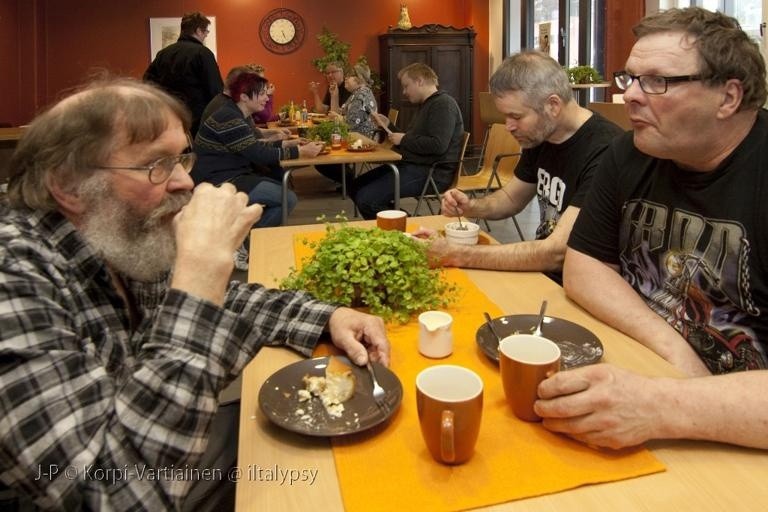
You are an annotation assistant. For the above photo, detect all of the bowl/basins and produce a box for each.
[445,220,480,250]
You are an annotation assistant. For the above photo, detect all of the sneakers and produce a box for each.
[235,244,249,271]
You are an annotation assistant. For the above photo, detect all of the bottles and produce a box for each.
[278,100,312,126]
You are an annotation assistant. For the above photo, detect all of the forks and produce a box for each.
[364,353,392,420]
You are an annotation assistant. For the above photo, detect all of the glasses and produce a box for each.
[613,71,715,95]
[89,152,195,185]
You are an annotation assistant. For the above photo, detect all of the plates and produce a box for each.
[312,142,376,154]
[437,230,491,246]
[478,312,603,374]
[257,356,401,437]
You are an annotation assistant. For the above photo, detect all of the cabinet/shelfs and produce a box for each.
[378,23,478,159]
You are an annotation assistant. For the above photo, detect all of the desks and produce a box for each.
[569,77,612,109]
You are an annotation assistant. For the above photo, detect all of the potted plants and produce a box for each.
[562,64,603,84]
[273,206,460,325]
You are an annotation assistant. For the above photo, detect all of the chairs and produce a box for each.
[610,93,627,104]
[457,123,527,242]
[404,131,471,217]
[588,101,634,131]
[352,109,399,215]
[476,90,509,177]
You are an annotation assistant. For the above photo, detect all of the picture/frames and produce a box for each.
[148,16,218,67]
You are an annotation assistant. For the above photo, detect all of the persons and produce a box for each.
[531,5,768,453]
[140,11,467,276]
[407,50,627,286]
[1,78,392,512]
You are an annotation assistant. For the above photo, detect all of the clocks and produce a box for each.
[258,8,306,55]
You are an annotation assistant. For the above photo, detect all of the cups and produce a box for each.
[500,334,561,427]
[417,310,454,358]
[417,362,487,462]
[331,116,341,150]
[376,210,408,232]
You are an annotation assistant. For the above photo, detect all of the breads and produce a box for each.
[297,356,355,407]
